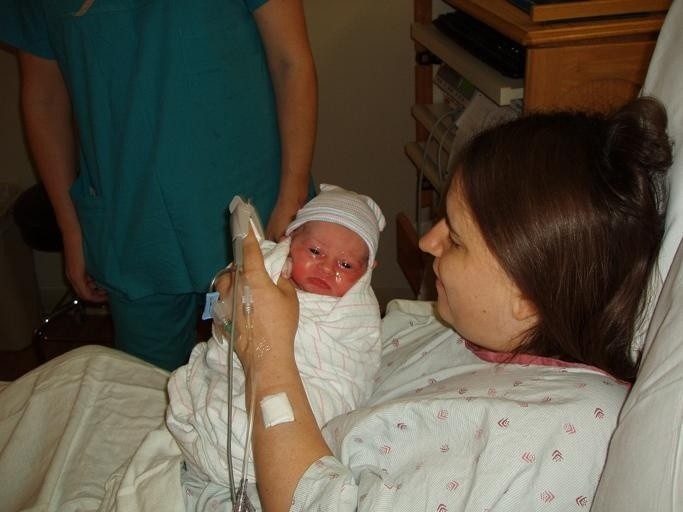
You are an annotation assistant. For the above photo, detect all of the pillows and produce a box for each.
[589,2,680,509]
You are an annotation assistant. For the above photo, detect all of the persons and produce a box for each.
[0,1,319,372]
[166,184,387,487]
[1,97,674,512]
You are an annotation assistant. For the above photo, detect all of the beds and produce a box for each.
[2,0,681,509]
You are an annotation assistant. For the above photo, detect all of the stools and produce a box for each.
[9,181,113,362]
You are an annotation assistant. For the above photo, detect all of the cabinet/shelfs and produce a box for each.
[396,0,672,302]
